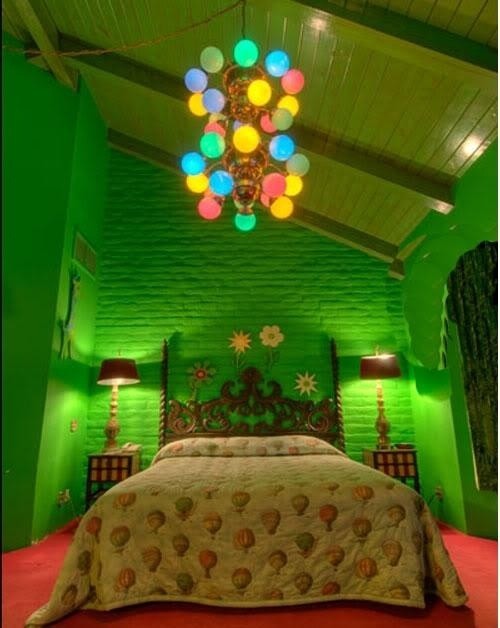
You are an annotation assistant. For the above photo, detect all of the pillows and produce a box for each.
[151,435,346,465]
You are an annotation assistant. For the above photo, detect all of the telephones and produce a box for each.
[122,443,141,452]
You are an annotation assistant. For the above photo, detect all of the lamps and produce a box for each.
[179,1,311,233]
[96,359,141,453]
[360,354,401,449]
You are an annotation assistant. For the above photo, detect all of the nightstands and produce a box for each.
[363,446,421,495]
[85,451,141,512]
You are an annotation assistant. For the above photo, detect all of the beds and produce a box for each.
[23,336,469,628]
